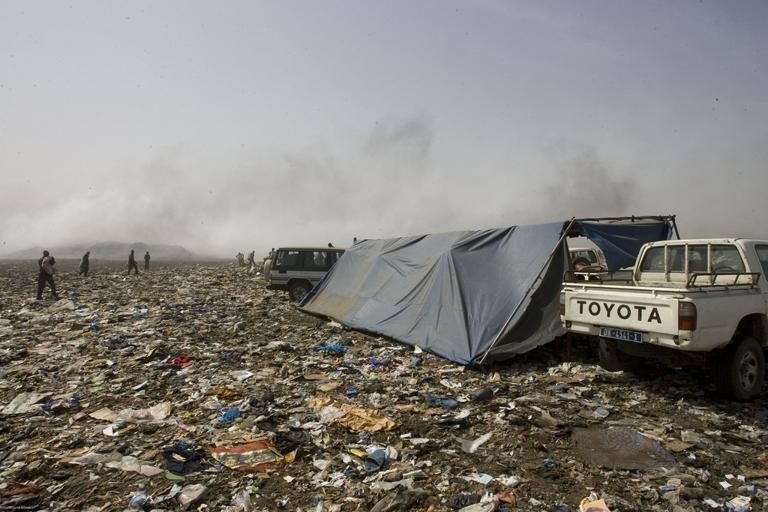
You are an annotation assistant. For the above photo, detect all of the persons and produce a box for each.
[316,251,325,265]
[235,251,247,267]
[127,249,140,275]
[327,242,335,248]
[37,250,62,301]
[143,250,151,270]
[268,247,275,257]
[247,250,256,267]
[79,251,92,278]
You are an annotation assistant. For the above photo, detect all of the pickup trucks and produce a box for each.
[559,238,767,404]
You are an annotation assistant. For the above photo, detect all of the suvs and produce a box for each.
[566,247,605,268]
[262,245,344,303]
